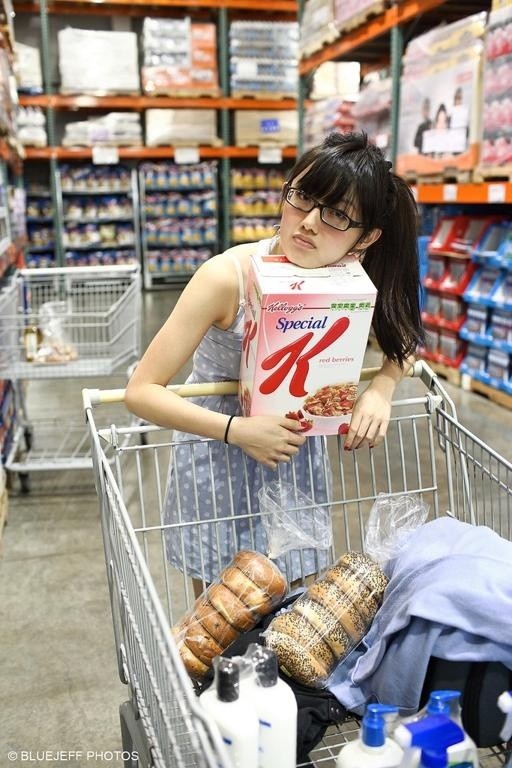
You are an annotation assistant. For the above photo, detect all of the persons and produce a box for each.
[449,88,468,139]
[435,105,449,128]
[125,131,425,603]
[414,100,434,155]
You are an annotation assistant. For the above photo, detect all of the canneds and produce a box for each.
[25,327,42,362]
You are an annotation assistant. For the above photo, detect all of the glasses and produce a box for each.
[284,181,365,232]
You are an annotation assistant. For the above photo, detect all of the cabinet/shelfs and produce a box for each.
[1,1,299,286]
[300,0,512,408]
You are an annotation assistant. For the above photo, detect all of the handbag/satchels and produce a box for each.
[197,592,360,763]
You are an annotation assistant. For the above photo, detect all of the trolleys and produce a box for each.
[83,359,511,767]
[1,268,140,494]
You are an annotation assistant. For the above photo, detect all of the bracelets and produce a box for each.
[224,415,236,444]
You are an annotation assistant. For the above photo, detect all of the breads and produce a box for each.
[266,550,389,687]
[170,548,289,677]
